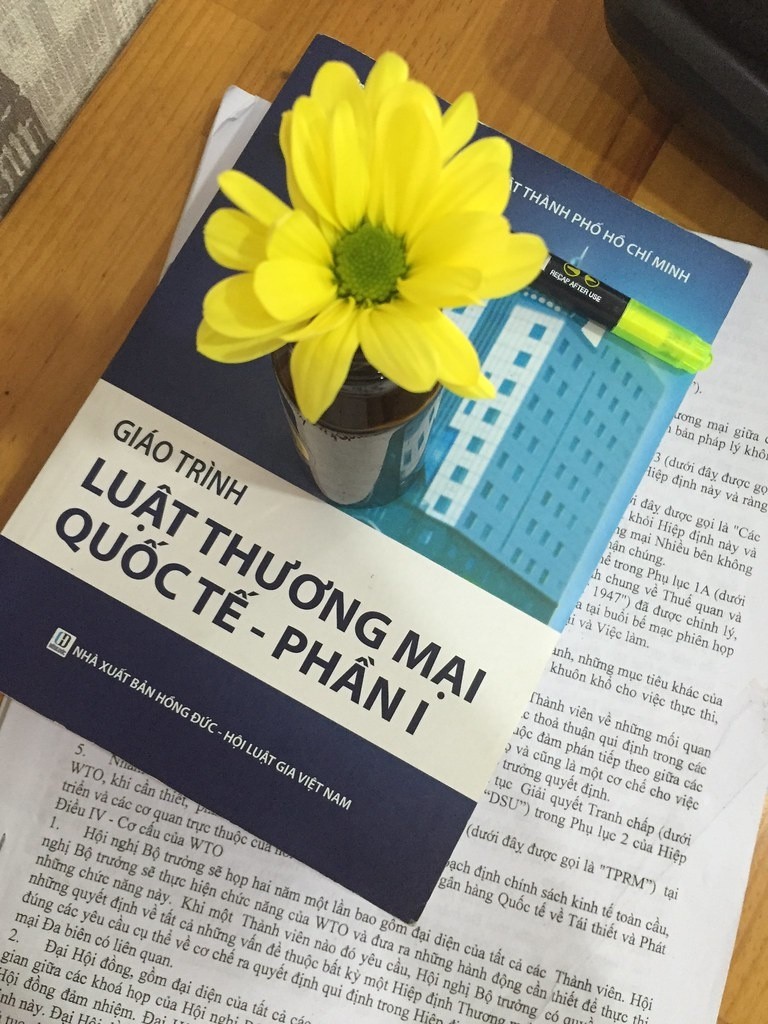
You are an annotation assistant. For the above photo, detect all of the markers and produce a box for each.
[510,229,714,375]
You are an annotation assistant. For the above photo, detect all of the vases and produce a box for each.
[270,339,443,507]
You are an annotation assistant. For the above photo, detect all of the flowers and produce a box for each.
[192,46,551,421]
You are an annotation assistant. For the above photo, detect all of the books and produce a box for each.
[0,34,751,925]
